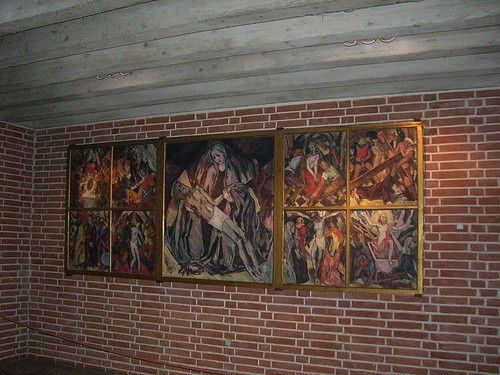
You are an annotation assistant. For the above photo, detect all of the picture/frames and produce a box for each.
[65,121,423,296]
